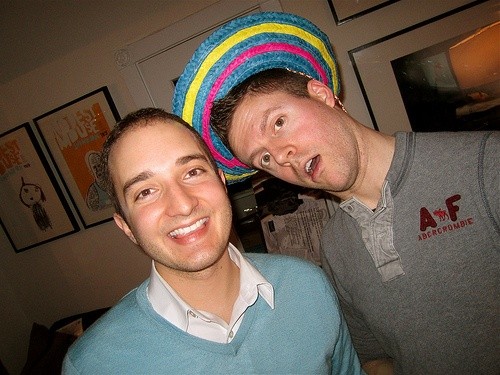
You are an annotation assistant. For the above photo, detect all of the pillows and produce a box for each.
[22,322,78,375]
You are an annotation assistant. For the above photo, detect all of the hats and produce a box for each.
[171,13,342,185]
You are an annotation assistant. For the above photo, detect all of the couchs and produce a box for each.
[20,306,112,375]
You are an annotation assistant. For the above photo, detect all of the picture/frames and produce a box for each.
[112,0,284,114]
[325,0,401,26]
[347,0,500,135]
[0,122,82,255]
[31,86,122,229]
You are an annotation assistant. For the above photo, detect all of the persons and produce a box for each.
[63,108,362,375]
[174,11,500,375]
